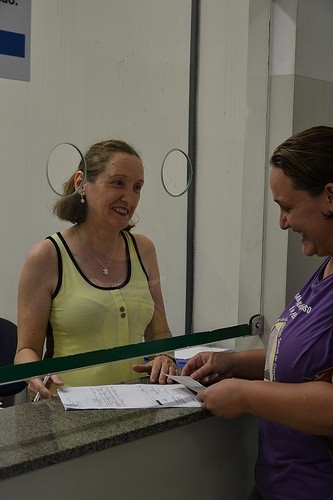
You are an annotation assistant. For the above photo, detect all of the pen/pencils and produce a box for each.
[33,374,50,403]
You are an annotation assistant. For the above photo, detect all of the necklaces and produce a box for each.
[90,244,118,275]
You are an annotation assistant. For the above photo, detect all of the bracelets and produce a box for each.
[152,349,178,364]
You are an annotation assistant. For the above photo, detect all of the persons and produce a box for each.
[181,125,333,500]
[14,140,180,402]
